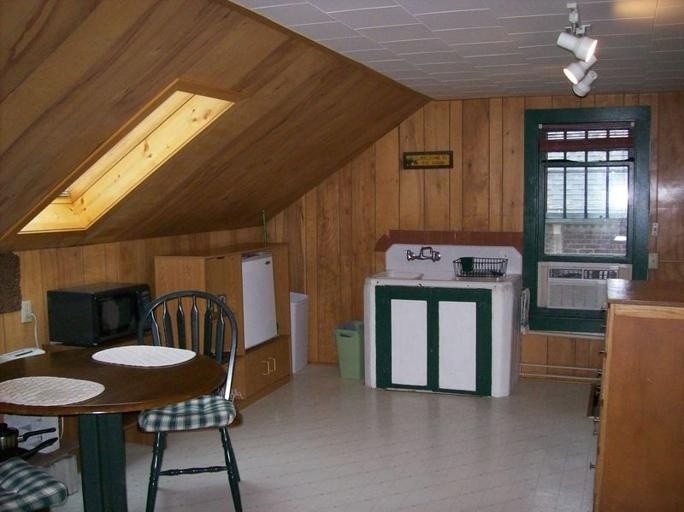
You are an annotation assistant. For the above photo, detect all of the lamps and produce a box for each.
[557,5,597,98]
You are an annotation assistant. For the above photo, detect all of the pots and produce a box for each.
[0,422,58,462]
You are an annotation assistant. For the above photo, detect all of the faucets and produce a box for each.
[406,246,441,263]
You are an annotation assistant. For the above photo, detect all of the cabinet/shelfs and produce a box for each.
[155,242,294,409]
[588,300,682,510]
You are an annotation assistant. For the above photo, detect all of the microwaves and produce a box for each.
[46,280,154,347]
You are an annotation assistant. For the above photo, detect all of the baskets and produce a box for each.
[453,257,508,277]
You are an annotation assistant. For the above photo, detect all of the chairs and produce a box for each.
[138,288,243,511]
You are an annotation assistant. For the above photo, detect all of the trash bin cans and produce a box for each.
[334,324,362,379]
[289,292,309,374]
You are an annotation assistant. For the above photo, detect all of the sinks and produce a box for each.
[375,271,424,280]
[417,271,456,281]
